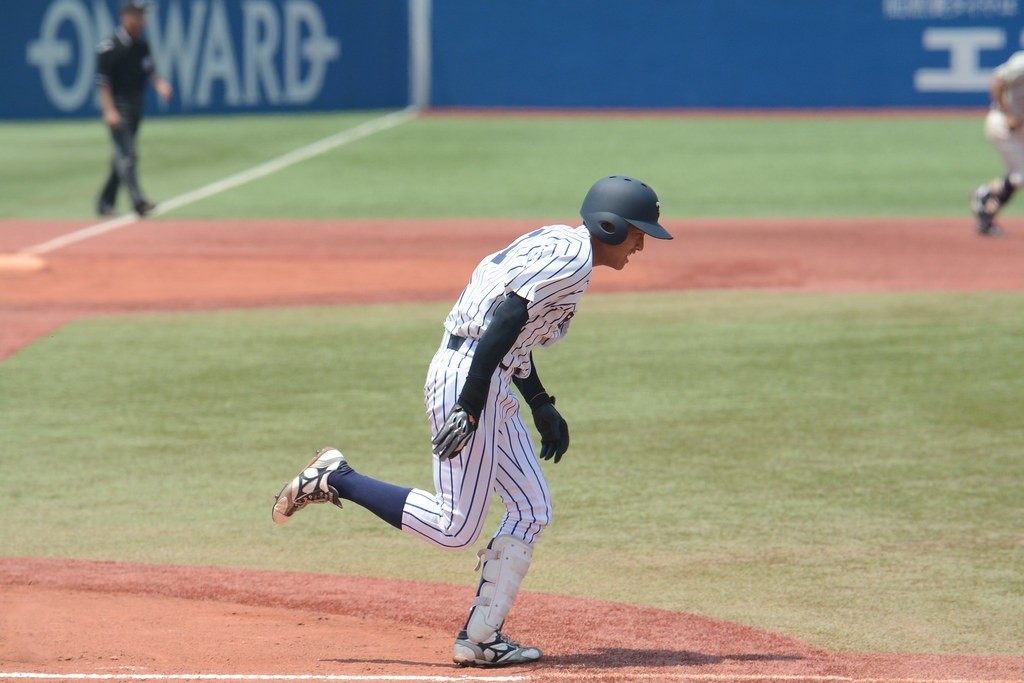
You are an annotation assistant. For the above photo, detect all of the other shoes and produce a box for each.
[137,202,159,215]
[976,176,1014,234]
[99,204,113,215]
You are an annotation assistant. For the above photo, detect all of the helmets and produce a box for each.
[580,176,674,244]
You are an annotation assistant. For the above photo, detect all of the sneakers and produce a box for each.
[453,629,543,670]
[272,446,346,526]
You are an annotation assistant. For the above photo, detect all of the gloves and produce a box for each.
[431,402,478,461]
[529,392,570,464]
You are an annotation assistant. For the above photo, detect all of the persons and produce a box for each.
[95,1,173,216]
[967,29,1024,237]
[272,176,674,666]
